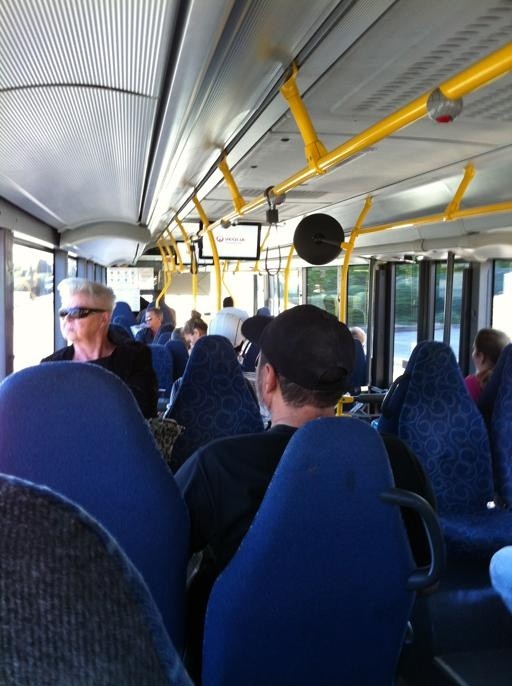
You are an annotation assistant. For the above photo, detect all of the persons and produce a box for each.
[132,307,167,344]
[206,295,251,354]
[235,307,273,376]
[166,301,441,685]
[346,326,368,350]
[39,276,160,427]
[462,327,511,410]
[182,310,209,357]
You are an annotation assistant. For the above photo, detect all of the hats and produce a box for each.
[241,303,356,392]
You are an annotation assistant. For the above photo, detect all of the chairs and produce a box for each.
[0,302,512,685]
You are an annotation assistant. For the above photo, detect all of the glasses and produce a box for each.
[58,306,106,320]
